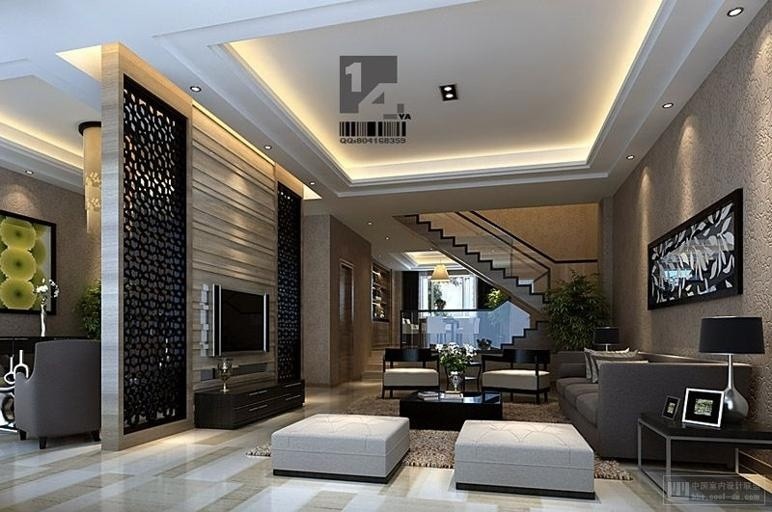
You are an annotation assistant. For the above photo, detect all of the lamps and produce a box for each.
[593,326,622,352]
[700,315,766,423]
[430,263,451,282]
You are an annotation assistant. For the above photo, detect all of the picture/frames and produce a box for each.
[0,208,59,316]
[682,387,725,427]
[661,395,681,421]
[646,187,744,310]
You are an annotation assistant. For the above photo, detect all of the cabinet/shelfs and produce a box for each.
[371,260,393,350]
[194,379,308,430]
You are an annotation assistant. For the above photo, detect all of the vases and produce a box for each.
[448,369,465,393]
[40,304,47,337]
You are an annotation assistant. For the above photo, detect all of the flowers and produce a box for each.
[35,277,60,305]
[436,343,478,371]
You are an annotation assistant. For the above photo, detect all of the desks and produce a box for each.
[419,317,459,346]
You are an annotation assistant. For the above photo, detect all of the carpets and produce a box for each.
[245,392,632,481]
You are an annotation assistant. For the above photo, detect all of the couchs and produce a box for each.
[550,351,753,469]
[381,347,440,398]
[270,412,410,484]
[479,347,552,405]
[452,418,595,502]
[14,338,102,448]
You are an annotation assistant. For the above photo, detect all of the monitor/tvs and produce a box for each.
[213,284,271,359]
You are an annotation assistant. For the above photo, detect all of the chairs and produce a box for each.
[427,316,447,343]
[456,317,480,343]
[402,317,425,346]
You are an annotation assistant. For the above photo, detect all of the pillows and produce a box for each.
[585,349,642,384]
[583,348,630,380]
